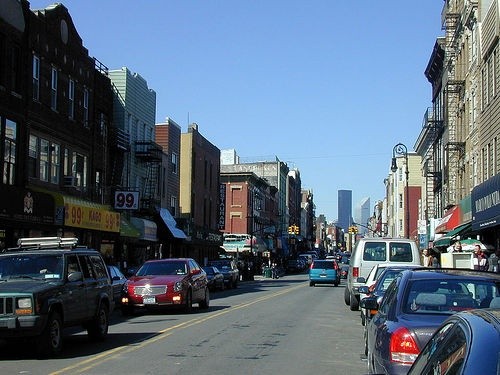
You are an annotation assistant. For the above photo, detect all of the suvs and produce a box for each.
[0,234,116,357]
[206,259,240,291]
[285,256,306,274]
[121,257,210,313]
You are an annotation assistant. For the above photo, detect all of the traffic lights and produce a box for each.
[288,225,293,234]
[354,226,359,234]
[348,225,353,234]
[294,225,299,235]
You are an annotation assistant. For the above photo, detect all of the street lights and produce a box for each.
[372,203,382,237]
[390,143,410,239]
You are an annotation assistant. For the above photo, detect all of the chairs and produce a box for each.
[414,293,500,313]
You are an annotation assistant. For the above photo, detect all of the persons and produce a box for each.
[473,245,489,271]
[448,241,463,253]
[420,248,433,267]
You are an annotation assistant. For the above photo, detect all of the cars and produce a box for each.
[357,262,426,326]
[307,258,342,287]
[335,252,345,262]
[324,255,336,259]
[340,265,349,279]
[357,264,429,326]
[342,254,352,264]
[299,253,312,266]
[307,251,320,260]
[405,306,500,375]
[359,267,500,375]
[107,264,128,305]
[202,266,226,292]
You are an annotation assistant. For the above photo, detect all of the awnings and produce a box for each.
[432,224,472,246]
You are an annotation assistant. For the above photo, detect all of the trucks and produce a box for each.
[343,236,422,312]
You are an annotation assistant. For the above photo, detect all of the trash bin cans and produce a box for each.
[264,267,270,278]
